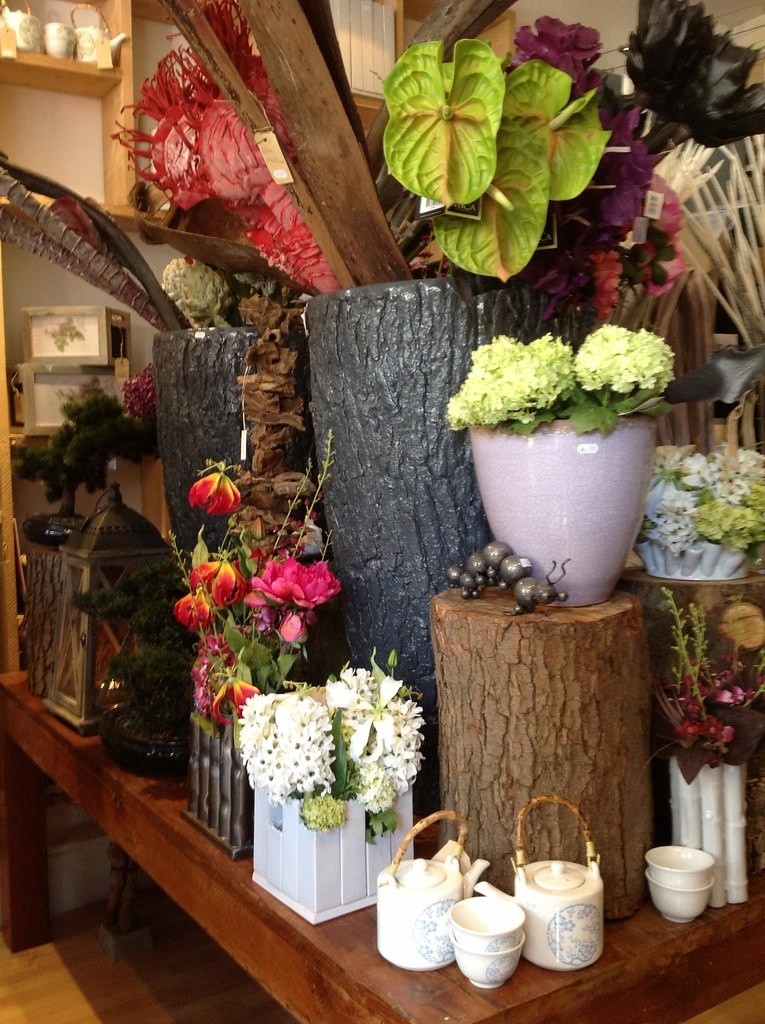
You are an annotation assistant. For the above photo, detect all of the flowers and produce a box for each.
[166,427,342,736]
[109,0,765,322]
[446,323,677,439]
[238,649,426,845]
[636,442,765,566]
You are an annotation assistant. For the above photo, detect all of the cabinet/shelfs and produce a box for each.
[0,0,518,674]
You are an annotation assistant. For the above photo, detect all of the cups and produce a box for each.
[646,846,715,888]
[645,868,715,923]
[450,930,525,989]
[449,897,525,952]
[44,23,75,58]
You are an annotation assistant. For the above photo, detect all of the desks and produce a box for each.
[0,670,765,1024]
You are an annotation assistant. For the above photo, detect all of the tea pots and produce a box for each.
[0,0,45,52]
[377,811,489,972]
[70,5,128,62]
[476,795,605,971]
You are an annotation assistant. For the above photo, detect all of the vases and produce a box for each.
[250,771,414,926]
[152,331,321,567]
[180,684,254,863]
[471,417,664,606]
[636,540,748,908]
[304,276,584,796]
